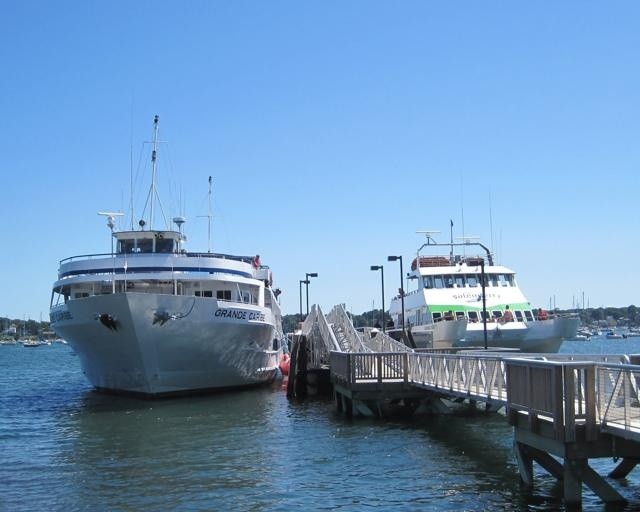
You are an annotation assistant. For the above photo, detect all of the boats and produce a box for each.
[45,89,286,399]
[1,337,65,350]
[389,216,579,355]
[539,289,639,341]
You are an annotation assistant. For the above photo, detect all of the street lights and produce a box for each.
[299,278,311,322]
[386,253,408,350]
[305,271,318,317]
[371,264,385,348]
[464,256,488,351]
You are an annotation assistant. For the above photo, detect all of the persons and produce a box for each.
[253,254,260,268]
[444,310,455,321]
[536,307,549,321]
[274,288,282,300]
[499,305,513,322]
